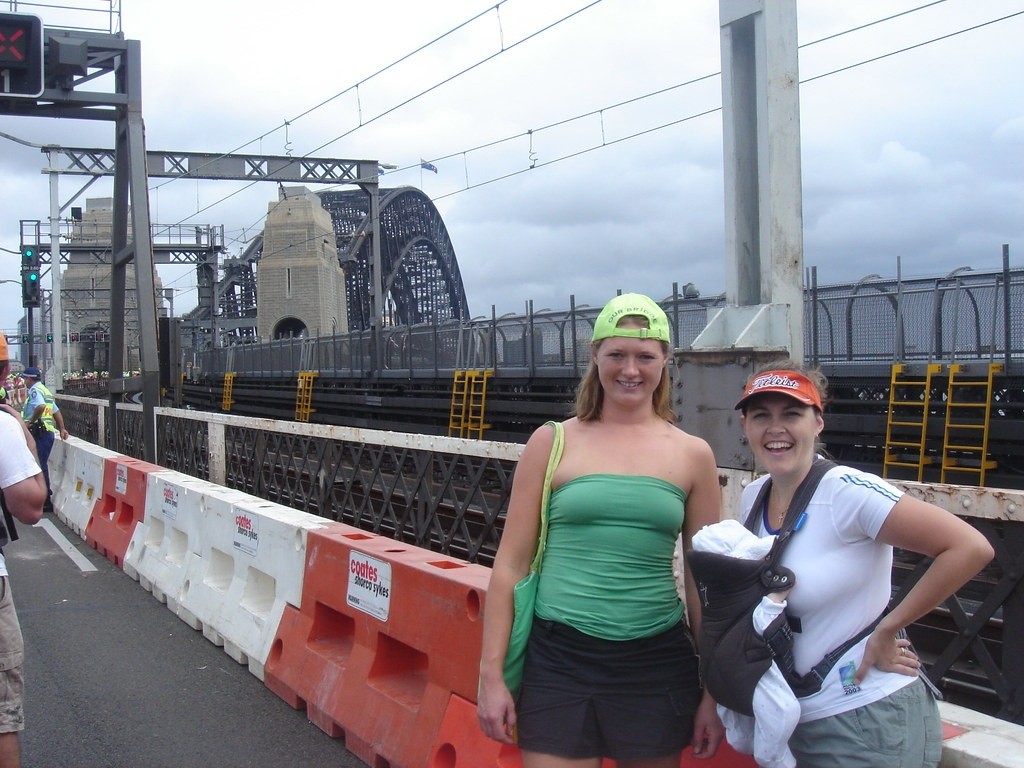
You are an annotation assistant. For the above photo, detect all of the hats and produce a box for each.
[733,372,823,415]
[0,333,10,362]
[19,366,41,377]
[592,292,671,343]
[692,519,777,561]
[0,386,6,399]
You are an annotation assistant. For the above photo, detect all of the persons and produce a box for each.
[0,331,69,768]
[691,358,994,768]
[478,294,725,768]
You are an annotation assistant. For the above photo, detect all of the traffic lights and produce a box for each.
[95,331,105,342]
[45,332,53,343]
[70,332,80,343]
[23,247,37,266]
[0,11,45,99]
[21,333,29,344]
[26,272,41,296]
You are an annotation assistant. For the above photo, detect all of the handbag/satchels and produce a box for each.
[28,421,46,436]
[477,422,565,728]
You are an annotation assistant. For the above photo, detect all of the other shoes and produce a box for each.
[43,503,53,513]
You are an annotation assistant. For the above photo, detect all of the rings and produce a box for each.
[901,647,905,655]
